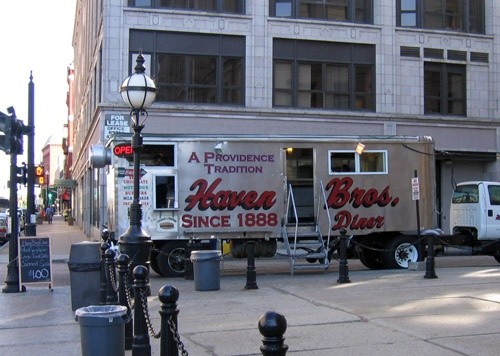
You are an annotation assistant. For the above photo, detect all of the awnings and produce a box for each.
[54,179,77,189]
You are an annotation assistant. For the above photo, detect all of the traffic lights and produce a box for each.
[34,166,45,177]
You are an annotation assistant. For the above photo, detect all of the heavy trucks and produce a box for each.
[102,135,500,277]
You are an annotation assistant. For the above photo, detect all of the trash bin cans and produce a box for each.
[68,241,103,311]
[191,250,221,291]
[74,305,128,356]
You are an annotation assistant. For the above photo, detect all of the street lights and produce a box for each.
[118,46,158,296]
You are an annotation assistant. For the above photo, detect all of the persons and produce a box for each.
[35,204,54,224]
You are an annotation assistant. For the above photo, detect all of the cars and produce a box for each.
[0,206,28,240]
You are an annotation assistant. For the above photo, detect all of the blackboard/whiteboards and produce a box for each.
[18,237,52,284]
